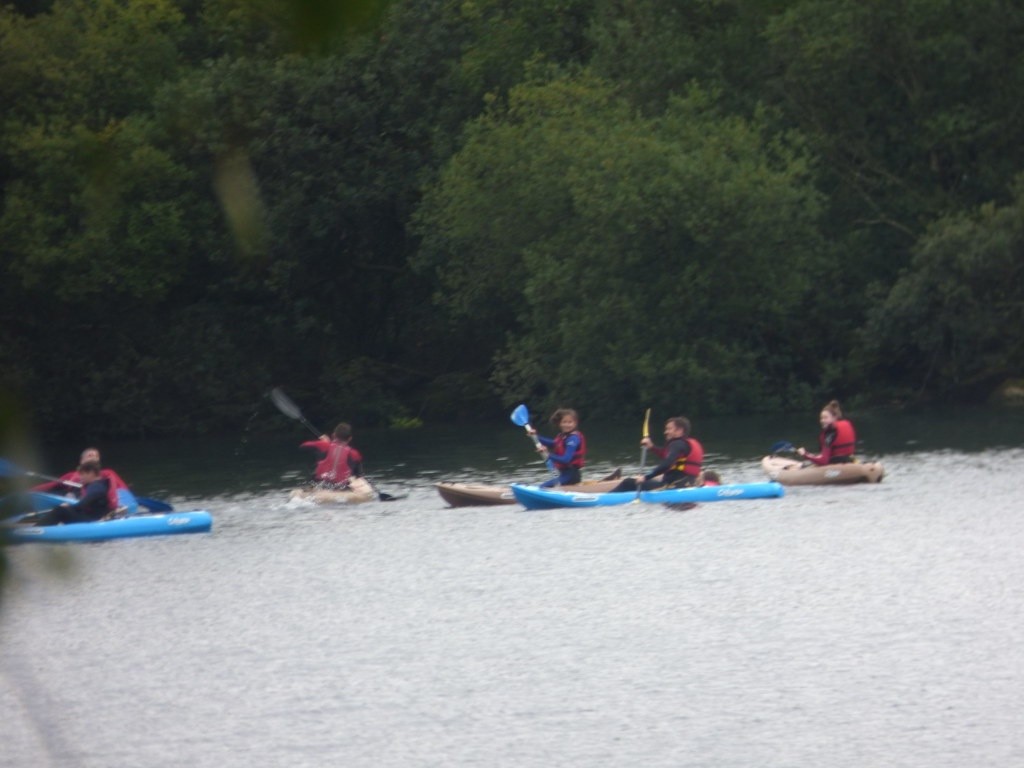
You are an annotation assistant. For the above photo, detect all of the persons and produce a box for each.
[301,423,365,490]
[527,409,586,488]
[30,448,131,527]
[609,418,704,492]
[797,400,856,466]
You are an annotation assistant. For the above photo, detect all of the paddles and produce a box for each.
[511,404,561,486]
[633,408,653,505]
[0,462,173,514]
[271,388,396,504]
[772,442,801,458]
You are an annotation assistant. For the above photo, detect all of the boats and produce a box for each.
[286,474,377,510]
[508,479,785,510]
[0,489,214,544]
[432,470,664,509]
[761,448,886,487]
